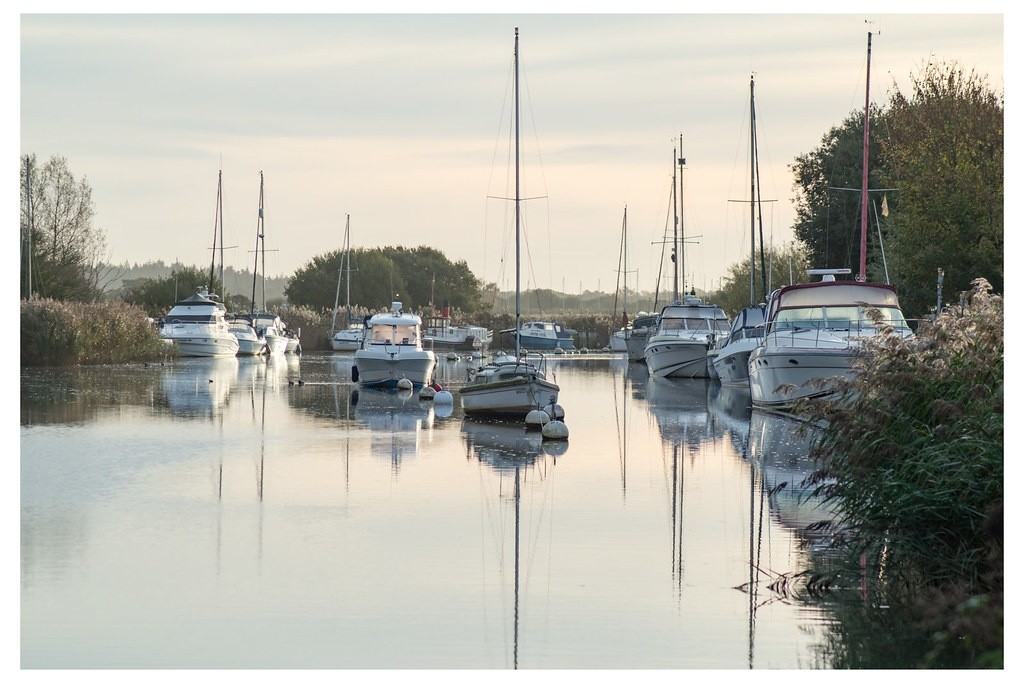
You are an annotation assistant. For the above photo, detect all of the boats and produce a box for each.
[510,320,578,347]
[349,300,440,389]
[419,272,494,351]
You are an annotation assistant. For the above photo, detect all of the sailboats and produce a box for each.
[331,211,365,352]
[157,167,303,360]
[459,24,560,417]
[608,30,923,408]
